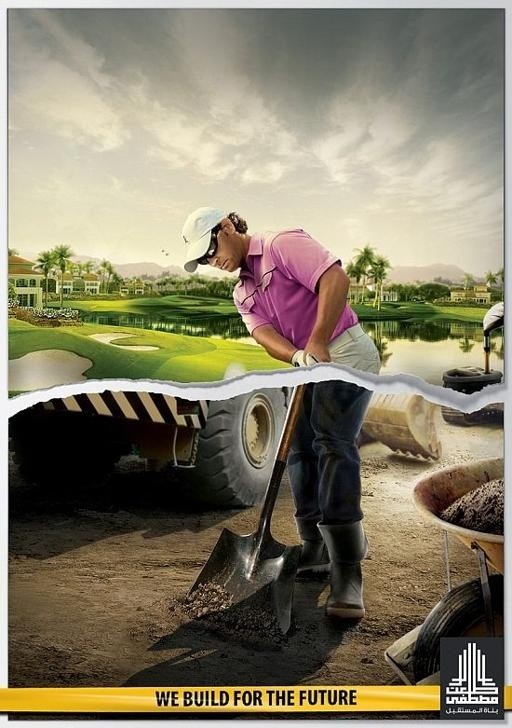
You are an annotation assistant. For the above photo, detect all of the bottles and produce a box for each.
[181,202,234,272]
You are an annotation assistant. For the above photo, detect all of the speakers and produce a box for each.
[315,518,365,618]
[292,512,333,574]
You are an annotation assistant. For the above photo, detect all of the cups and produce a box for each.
[196,225,223,266]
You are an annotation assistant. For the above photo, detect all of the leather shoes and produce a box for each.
[290,349,317,368]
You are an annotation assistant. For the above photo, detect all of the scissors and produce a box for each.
[184,356,319,636]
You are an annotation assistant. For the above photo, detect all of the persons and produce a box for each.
[182,206,384,621]
